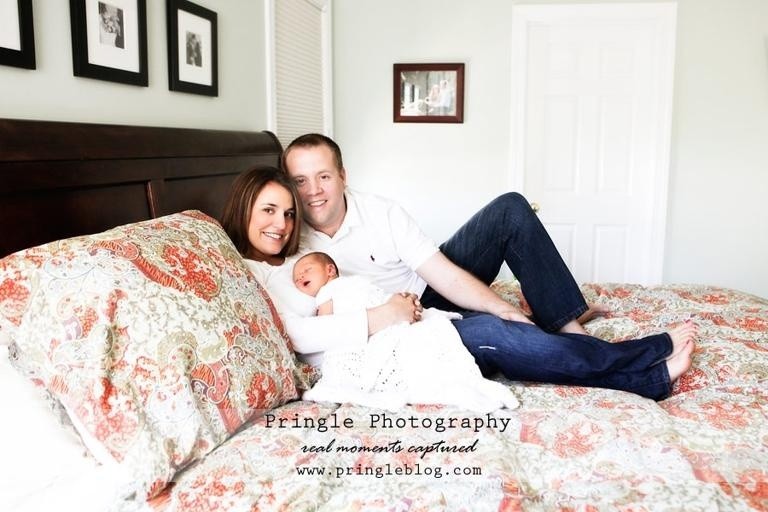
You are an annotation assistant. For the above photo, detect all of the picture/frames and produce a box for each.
[69,0,150,87]
[0,0,37,71]
[165,2,219,97]
[392,61,465,125]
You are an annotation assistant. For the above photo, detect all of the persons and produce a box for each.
[223,166,701,398]
[293,252,389,309]
[191,36,202,67]
[425,80,453,115]
[281,133,612,336]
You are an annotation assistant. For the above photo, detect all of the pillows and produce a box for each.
[0,207,324,502]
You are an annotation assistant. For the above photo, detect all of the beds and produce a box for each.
[0,119,767,510]
